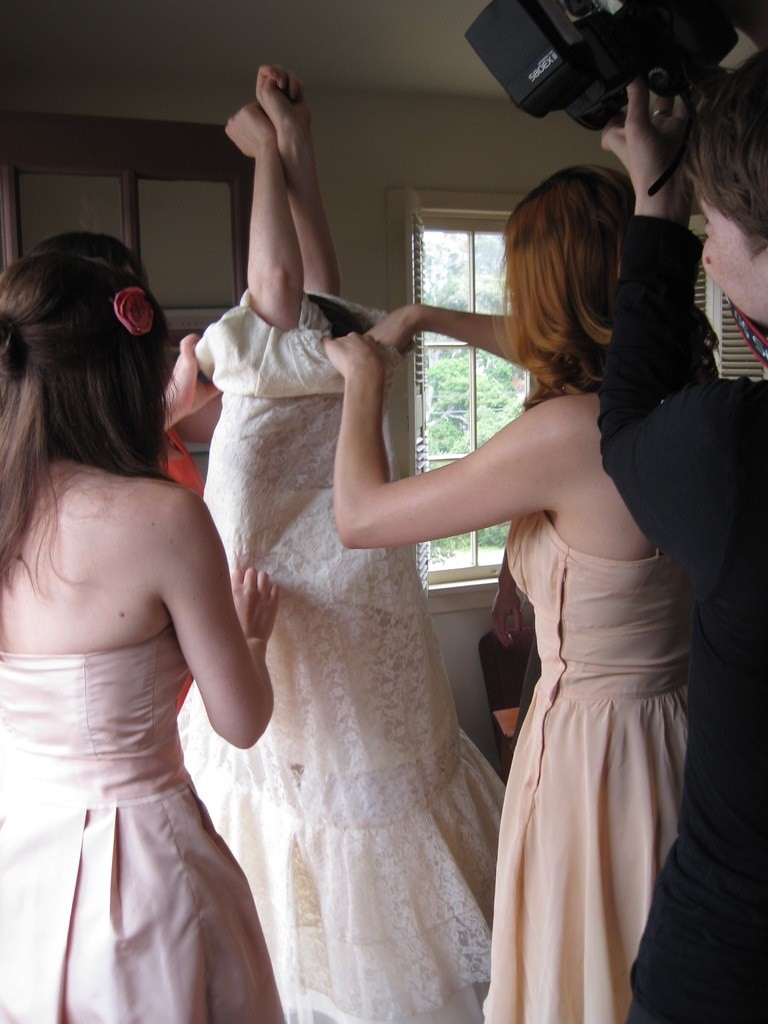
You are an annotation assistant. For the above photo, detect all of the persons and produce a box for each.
[571,66,768,1024]
[1,232,297,1024]
[173,58,527,1024]
[324,155,714,1024]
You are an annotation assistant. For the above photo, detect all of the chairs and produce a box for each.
[477,625,534,765]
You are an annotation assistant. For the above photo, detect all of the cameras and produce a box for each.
[465,0,737,124]
